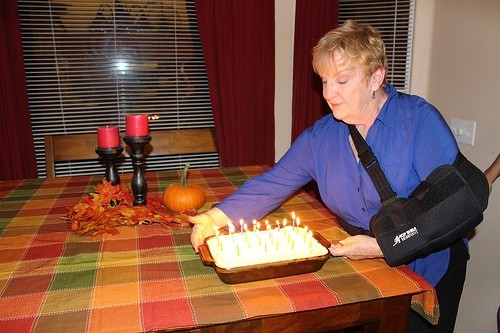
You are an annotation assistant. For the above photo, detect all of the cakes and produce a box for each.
[209,227,328,269]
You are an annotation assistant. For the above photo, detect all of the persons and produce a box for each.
[188,20,470,333]
[483,153,499,195]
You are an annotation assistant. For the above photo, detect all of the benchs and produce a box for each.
[45,128,220,178]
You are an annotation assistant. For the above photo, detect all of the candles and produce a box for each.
[125,113,149,137]
[97,125,120,149]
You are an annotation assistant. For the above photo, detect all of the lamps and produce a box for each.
[214,211,314,262]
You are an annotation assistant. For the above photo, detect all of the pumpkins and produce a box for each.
[164,164,205,212]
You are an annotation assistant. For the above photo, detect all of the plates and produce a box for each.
[198,229,332,284]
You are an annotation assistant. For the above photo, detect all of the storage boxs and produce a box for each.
[197,224,331,285]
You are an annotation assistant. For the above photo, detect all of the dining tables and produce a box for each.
[0,165,442,333]
